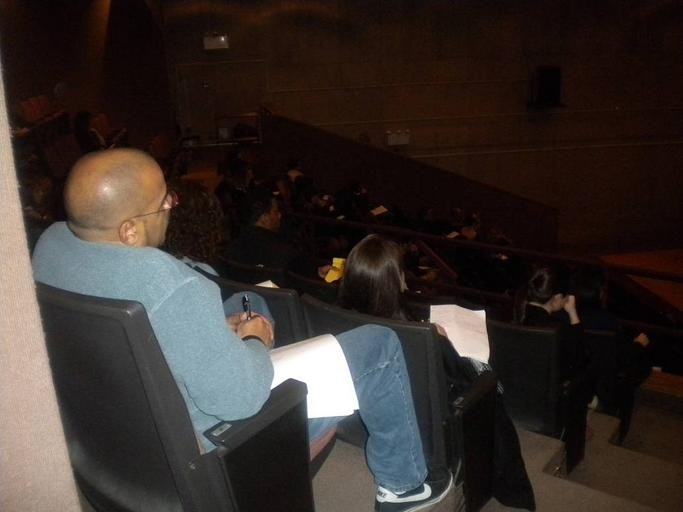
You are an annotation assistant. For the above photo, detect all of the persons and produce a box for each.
[31,146,454,510]
[337,182,657,512]
[7,112,337,310]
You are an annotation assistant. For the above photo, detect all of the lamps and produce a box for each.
[203,28,229,51]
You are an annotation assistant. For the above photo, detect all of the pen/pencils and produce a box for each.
[242,293,250,320]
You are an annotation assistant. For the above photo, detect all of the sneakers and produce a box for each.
[374,465,455,512]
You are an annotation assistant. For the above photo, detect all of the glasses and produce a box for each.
[130,189,180,221]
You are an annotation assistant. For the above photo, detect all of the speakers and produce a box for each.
[537,67,561,104]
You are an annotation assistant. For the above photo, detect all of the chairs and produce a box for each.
[9,94,562,512]
